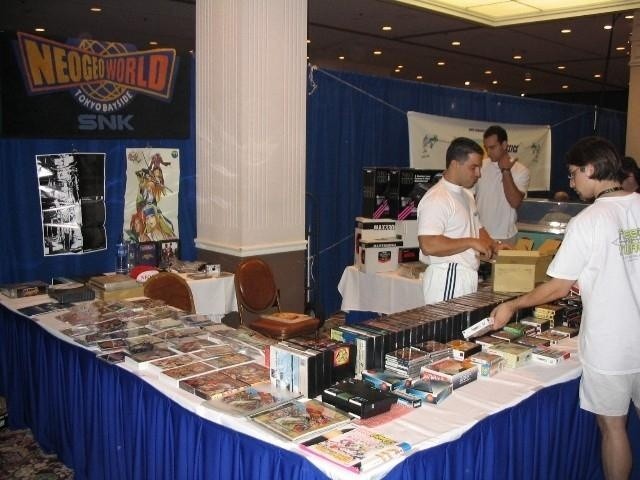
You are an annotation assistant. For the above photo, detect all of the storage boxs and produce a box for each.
[354,217,404,273]
[474,237,563,294]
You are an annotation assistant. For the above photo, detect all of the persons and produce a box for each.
[490,138,640,480]
[469,126,531,248]
[416,137,515,306]
[538,191,573,225]
[130,154,176,242]
[619,157,640,194]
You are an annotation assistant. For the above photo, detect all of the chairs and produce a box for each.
[143,256,321,343]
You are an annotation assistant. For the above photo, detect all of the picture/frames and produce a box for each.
[138,240,181,267]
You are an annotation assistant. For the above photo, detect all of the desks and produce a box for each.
[0,299,582,480]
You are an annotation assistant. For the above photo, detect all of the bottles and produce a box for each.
[116,241,129,275]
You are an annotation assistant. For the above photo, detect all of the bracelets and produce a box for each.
[497,240,503,244]
[501,168,510,173]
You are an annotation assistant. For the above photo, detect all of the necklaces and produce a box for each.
[596,186,625,198]
